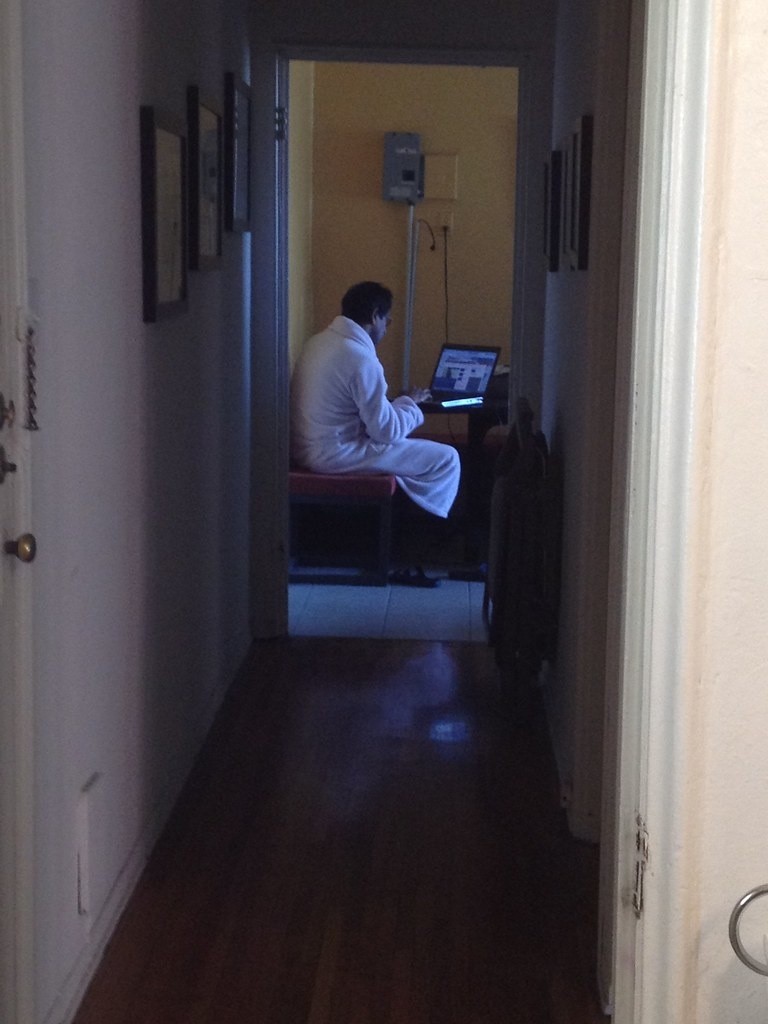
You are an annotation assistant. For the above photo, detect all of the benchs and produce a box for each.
[288,466,396,586]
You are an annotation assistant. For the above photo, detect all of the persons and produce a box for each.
[290,281,460,590]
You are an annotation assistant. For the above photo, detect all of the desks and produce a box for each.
[390,395,506,578]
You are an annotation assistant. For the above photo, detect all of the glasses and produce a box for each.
[381,314,396,326]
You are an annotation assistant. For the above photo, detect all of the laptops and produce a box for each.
[415,343,501,407]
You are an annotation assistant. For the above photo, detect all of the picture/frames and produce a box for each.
[141,104,189,321]
[541,150,558,271]
[563,115,591,267]
[188,85,226,267]
[223,72,255,229]
